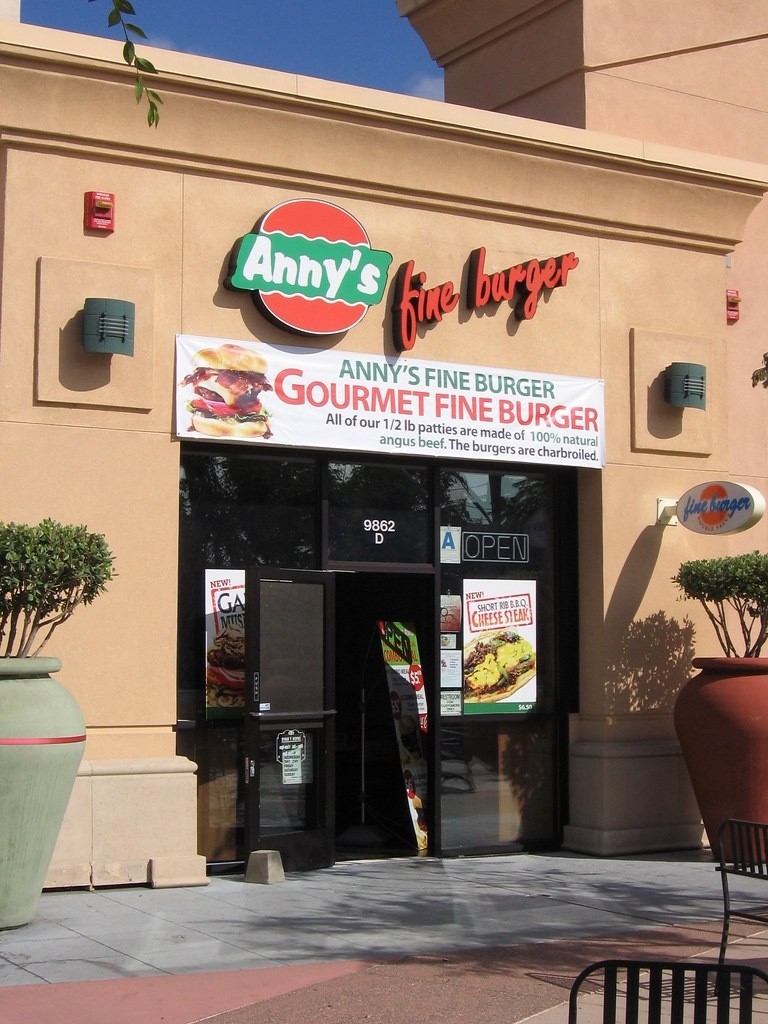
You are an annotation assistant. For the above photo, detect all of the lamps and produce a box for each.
[82,298,135,357]
[664,362,708,410]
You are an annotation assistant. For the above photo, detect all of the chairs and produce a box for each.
[568,960,768,1024]
[714,819,768,965]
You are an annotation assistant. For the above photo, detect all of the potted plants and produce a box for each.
[668,549,768,865]
[0,518,120,930]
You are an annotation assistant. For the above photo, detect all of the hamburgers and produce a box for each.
[177,342,274,441]
